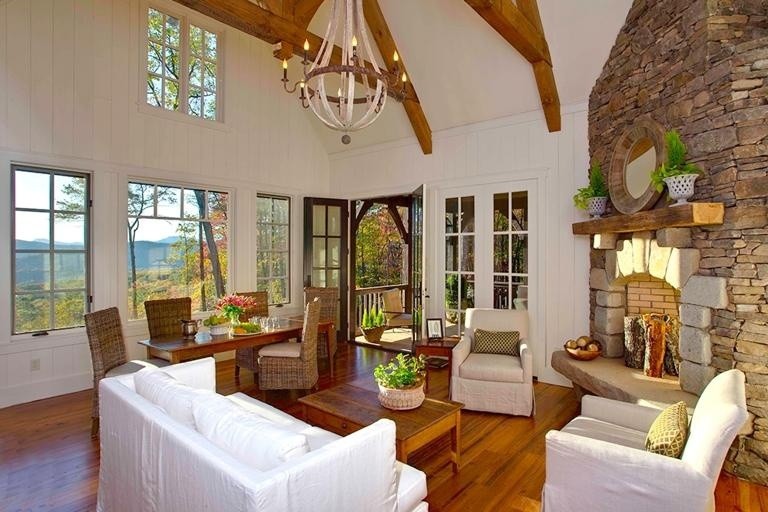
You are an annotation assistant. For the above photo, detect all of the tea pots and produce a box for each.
[177,318,202,341]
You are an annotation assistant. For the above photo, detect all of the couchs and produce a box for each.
[94,356,436,512]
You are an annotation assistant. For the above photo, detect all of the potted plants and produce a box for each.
[359,304,388,344]
[372,352,430,411]
[573,158,611,221]
[649,128,704,210]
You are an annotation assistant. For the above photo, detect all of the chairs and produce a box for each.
[254,293,325,404]
[535,366,751,510]
[379,286,416,333]
[232,290,291,386]
[82,304,172,438]
[142,296,212,367]
[446,306,536,419]
[285,284,340,363]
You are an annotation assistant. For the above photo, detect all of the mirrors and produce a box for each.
[605,118,672,215]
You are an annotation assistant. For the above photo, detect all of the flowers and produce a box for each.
[212,295,257,322]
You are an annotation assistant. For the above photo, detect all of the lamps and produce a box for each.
[276,0,411,148]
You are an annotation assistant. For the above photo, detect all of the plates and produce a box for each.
[230,330,264,336]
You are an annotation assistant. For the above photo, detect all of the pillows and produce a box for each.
[647,397,690,458]
[470,327,521,357]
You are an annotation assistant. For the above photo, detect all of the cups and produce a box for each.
[248,315,280,330]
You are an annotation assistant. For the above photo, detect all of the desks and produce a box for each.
[136,318,338,384]
[410,338,463,382]
[294,379,463,492]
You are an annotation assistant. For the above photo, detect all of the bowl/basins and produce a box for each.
[563,345,602,360]
[209,325,229,334]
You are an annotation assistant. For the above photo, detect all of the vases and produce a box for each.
[237,313,250,323]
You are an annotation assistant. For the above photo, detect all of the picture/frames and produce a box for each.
[425,317,445,341]
